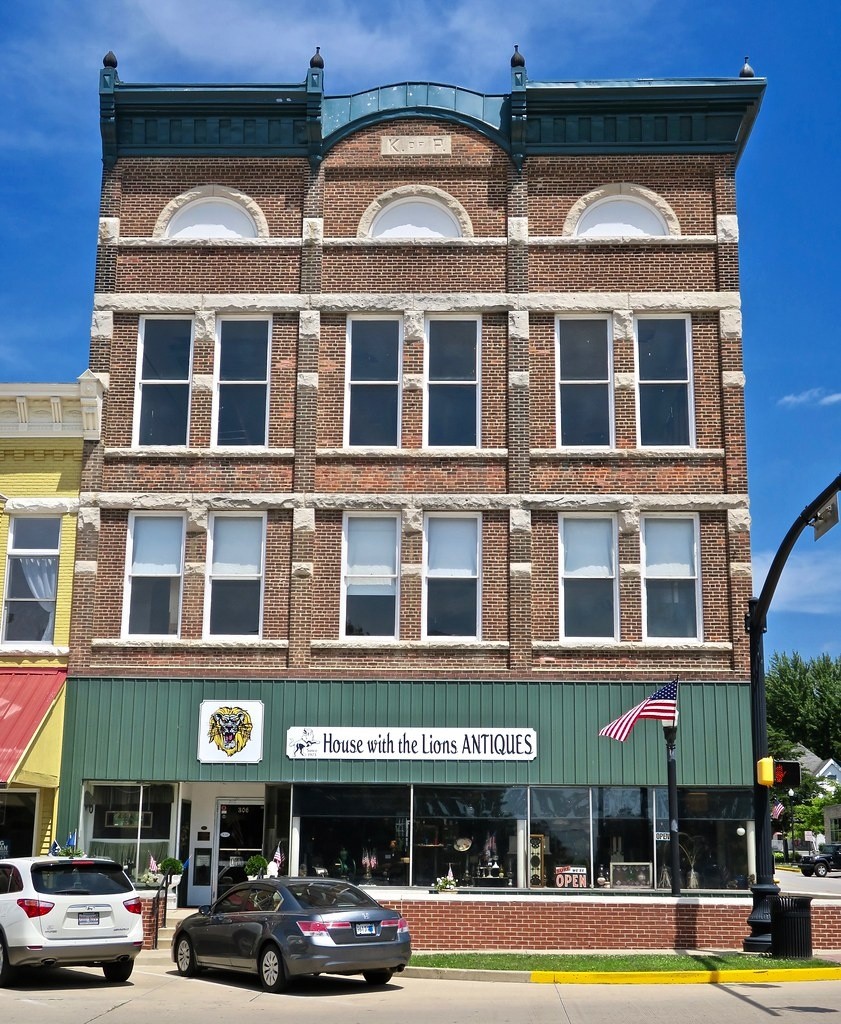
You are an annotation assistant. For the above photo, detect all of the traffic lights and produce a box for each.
[772,760,801,788]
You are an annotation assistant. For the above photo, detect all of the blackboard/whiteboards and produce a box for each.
[610,862,653,889]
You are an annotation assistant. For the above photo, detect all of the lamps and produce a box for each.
[736,823,745,836]
[85,790,102,814]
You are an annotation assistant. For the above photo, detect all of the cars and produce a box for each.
[171,876,411,993]
[217,860,252,895]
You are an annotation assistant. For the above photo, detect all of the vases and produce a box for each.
[437,891,458,894]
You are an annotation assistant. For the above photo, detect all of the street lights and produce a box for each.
[661,709,683,897]
[787,789,798,868]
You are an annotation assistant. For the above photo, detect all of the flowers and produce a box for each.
[431,877,459,891]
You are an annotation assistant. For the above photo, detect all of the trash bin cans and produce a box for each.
[766,895,813,960]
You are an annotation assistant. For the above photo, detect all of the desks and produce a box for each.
[472,877,508,887]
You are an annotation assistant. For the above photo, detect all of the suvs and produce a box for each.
[0,856,144,988]
[798,842,841,878]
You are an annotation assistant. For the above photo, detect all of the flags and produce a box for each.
[184,857,190,868]
[275,845,285,868]
[51,831,76,856]
[361,850,379,869]
[598,679,678,742]
[773,799,784,820]
[149,857,157,873]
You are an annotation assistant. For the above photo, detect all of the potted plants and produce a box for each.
[243,855,270,882]
[157,858,183,909]
